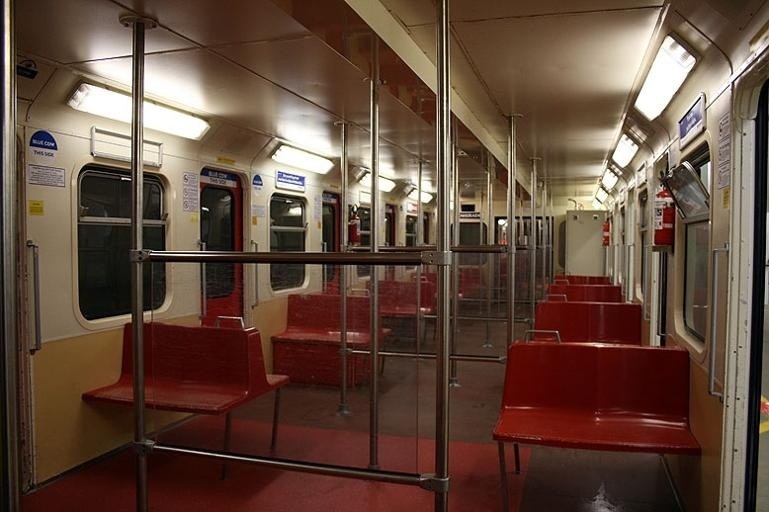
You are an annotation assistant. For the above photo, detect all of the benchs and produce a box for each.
[533,301,643,345]
[552,275,612,284]
[82,322,290,481]
[361,268,483,346]
[547,285,622,300]
[494,340,700,512]
[270,294,390,388]
